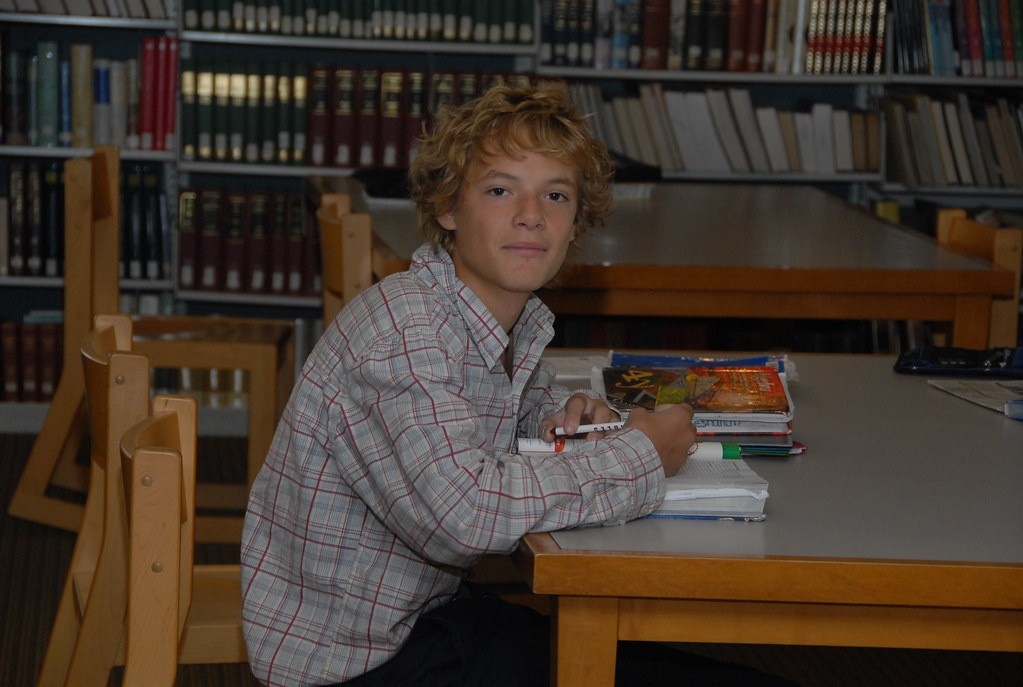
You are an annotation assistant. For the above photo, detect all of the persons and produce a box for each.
[238,95,697,687]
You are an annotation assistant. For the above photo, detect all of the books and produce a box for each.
[4,0,1022,521]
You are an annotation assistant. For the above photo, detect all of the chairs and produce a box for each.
[32,326,156,687]
[314,186,374,341]
[121,410,199,685]
[8,141,302,563]
[930,203,1023,351]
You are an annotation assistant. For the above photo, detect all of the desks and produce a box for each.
[366,181,1015,356]
[496,347,1023,682]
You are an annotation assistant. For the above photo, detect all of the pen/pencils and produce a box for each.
[548,422,626,436]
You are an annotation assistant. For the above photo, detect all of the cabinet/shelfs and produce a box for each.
[0,3,1023,444]
